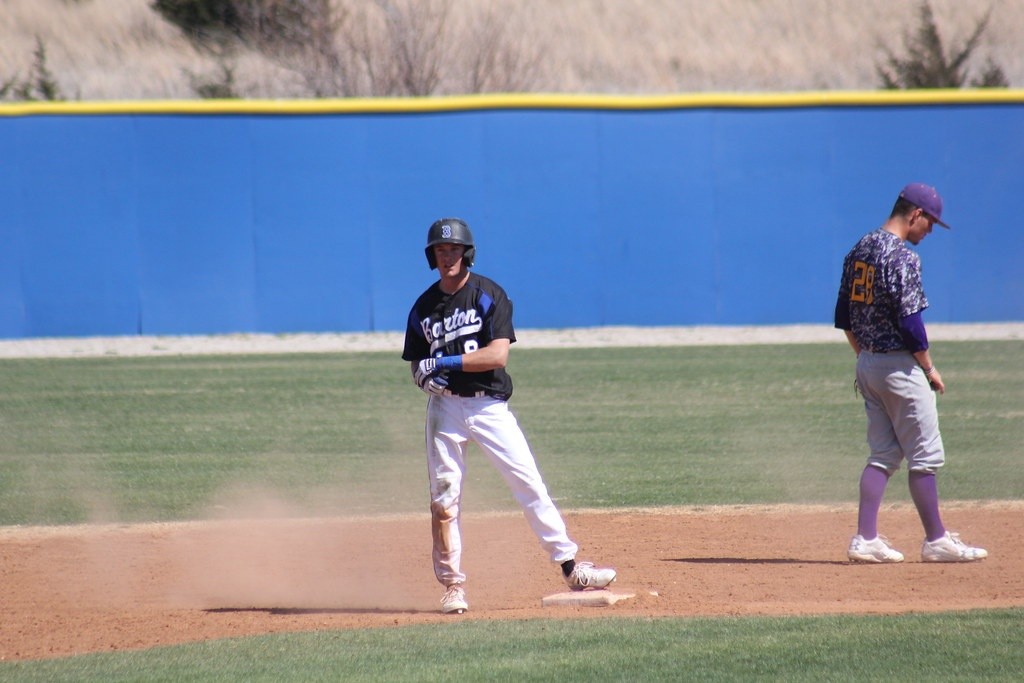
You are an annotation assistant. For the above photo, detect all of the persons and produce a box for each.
[402,218,616,615]
[834,183,987,563]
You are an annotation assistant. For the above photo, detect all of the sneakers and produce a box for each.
[921,530,988,562]
[563,562,616,591]
[846,533,904,562]
[442,587,468,614]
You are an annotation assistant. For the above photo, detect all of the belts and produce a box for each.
[444,389,487,398]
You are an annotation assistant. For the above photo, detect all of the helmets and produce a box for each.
[425,218,476,271]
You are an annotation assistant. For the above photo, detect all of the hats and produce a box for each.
[900,184,950,229]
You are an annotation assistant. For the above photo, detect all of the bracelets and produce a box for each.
[924,366,935,376]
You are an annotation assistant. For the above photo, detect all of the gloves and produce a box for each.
[415,358,437,387]
[414,373,449,397]
